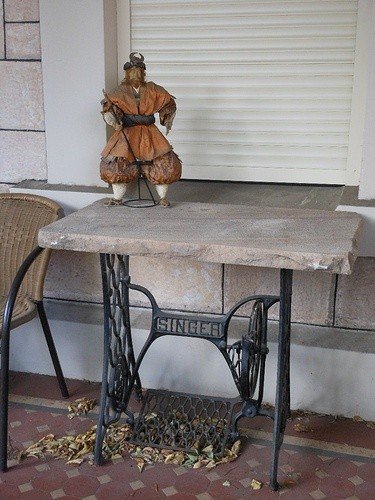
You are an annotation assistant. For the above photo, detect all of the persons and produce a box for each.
[100,52,183,207]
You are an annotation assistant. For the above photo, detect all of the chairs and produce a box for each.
[0,190,68,474]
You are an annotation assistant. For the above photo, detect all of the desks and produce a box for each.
[37,197,363,491]
